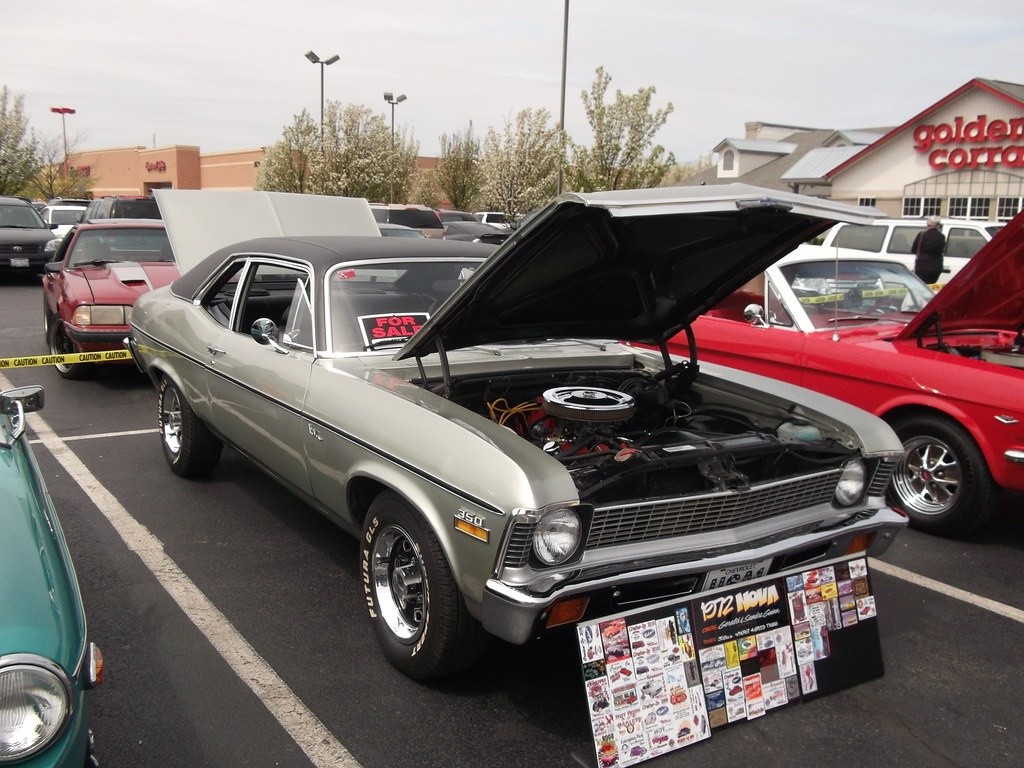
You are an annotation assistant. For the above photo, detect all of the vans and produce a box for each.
[820,217,1009,293]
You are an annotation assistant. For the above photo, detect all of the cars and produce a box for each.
[36,206,90,238]
[369,197,520,245]
[0,364,107,767]
[31,200,44,216]
[40,217,182,380]
[82,196,163,223]
[0,195,58,279]
[45,196,95,210]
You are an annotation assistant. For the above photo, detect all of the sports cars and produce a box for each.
[125,161,917,690]
[622,203,1024,533]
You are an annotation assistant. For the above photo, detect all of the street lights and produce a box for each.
[382,91,408,201]
[50,107,78,197]
[304,50,342,191]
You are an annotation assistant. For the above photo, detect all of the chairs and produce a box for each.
[80,242,115,261]
[708,290,764,323]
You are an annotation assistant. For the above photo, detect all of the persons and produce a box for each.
[911,215,946,284]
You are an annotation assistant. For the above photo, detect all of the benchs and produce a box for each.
[226,288,427,350]
